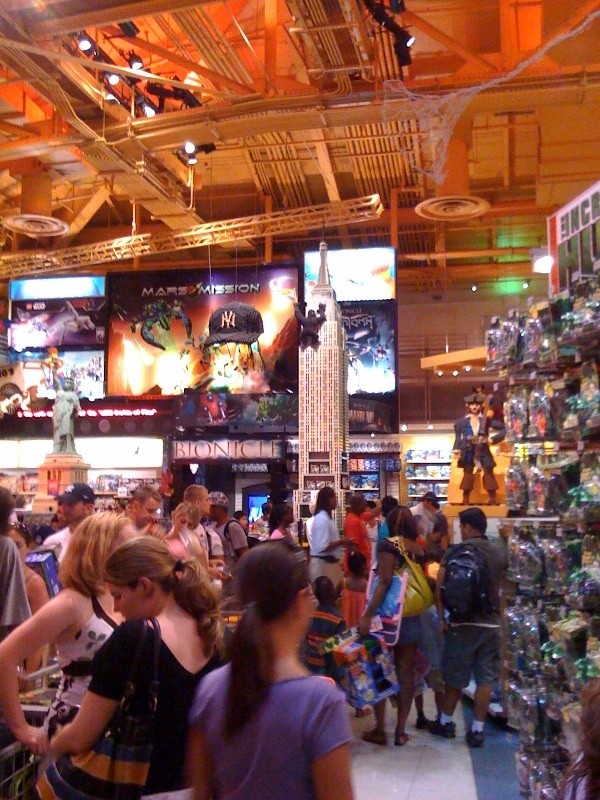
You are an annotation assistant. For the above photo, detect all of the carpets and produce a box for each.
[462,672,520,800]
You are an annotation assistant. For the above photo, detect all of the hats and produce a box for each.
[54,483,95,505]
[208,491,228,508]
[422,491,441,502]
[203,302,265,346]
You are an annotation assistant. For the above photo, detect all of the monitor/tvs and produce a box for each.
[247,494,270,523]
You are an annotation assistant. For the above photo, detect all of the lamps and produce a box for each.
[363,0,415,67]
[530,247,554,274]
[77,21,216,165]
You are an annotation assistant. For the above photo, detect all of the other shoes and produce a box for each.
[356,708,370,717]
[415,716,432,729]
[361,728,387,746]
[394,734,409,746]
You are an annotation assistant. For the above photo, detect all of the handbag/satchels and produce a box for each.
[363,536,435,645]
[35,616,160,800]
[223,520,261,557]
[347,540,367,576]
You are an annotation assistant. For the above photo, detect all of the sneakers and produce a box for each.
[466,729,485,747]
[427,715,456,739]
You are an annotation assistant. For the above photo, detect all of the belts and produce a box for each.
[312,554,325,559]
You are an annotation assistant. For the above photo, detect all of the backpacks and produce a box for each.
[439,542,494,620]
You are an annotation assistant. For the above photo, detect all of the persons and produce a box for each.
[453,394,506,505]
[29,535,220,800]
[186,540,356,799]
[0,512,139,800]
[0,482,506,745]
[51,364,82,455]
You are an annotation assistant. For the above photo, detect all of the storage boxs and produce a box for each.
[333,632,401,709]
[23,549,61,598]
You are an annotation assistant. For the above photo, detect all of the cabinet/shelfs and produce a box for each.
[0,467,162,524]
[282,452,451,531]
